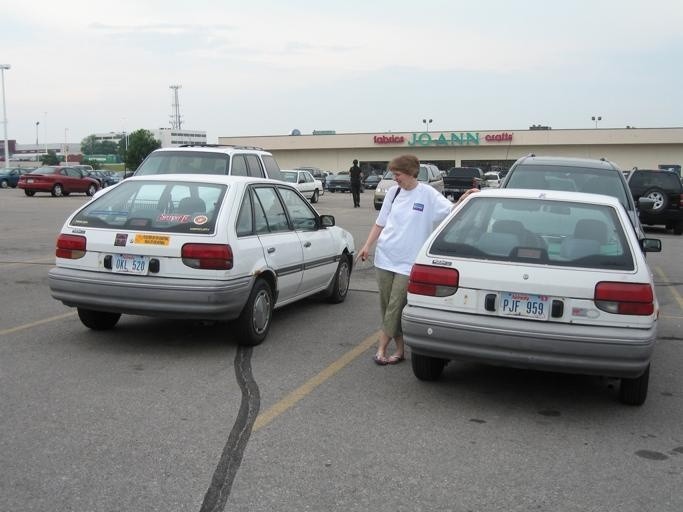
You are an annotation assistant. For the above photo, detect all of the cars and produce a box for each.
[400,188,661,405]
[46,173,356,347]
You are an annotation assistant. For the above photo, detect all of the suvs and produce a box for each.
[622,166,682,235]
[131,144,286,183]
[498,151,655,262]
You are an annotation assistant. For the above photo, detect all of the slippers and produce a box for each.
[374,353,386,366]
[388,355,406,366]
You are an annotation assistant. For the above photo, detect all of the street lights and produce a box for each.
[64,128,68,166]
[108,132,126,179]
[422,119,432,132]
[590,116,601,129]
[35,122,39,165]
[0,64,11,168]
[626,125,635,128]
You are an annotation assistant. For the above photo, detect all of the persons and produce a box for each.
[348,159,361,209]
[354,154,480,364]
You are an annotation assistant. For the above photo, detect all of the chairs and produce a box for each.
[474,231,516,258]
[560,219,610,257]
[491,219,526,247]
[560,236,601,261]
[175,196,205,216]
